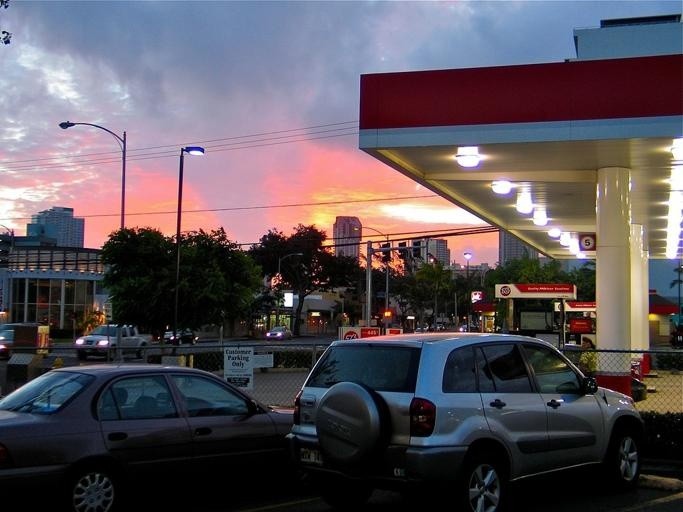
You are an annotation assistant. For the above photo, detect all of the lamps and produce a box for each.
[454,154,586,259]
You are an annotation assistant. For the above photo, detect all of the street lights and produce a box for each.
[172,146,207,353]
[464,252,472,328]
[353,225,390,330]
[59,120,127,232]
[278,252,303,326]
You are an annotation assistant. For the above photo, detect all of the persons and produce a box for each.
[577,337,598,373]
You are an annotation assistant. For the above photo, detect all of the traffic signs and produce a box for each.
[578,232,596,251]
